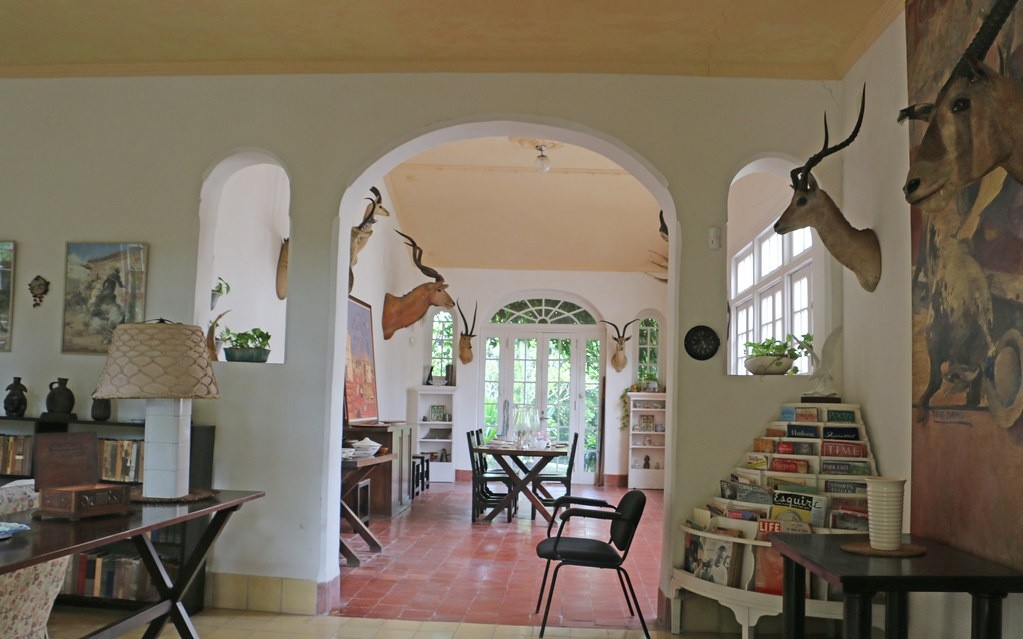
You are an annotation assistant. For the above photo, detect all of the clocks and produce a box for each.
[684,325,720,361]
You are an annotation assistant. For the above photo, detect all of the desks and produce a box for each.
[339,453,398,567]
[0,489,265,639]
[473,440,568,524]
[766,532,1023,639]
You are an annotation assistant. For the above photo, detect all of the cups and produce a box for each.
[444,414,449,421]
[534,440,548,449]
[864,475,908,551]
[550,437,556,450]
[423,416,427,421]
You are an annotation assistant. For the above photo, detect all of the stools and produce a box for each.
[414,453,430,490]
[411,459,422,499]
[353,478,371,527]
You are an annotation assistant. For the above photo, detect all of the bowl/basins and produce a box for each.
[428,380,447,385]
[341,444,388,458]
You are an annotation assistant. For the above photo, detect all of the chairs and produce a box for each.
[536,488,651,639]
[0,479,70,639]
[467,428,578,523]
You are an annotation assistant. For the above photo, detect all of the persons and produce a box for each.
[704,544,728,585]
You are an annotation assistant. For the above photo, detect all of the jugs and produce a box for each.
[440,448,447,462]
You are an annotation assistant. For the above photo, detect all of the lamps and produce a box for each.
[91,317,222,505]
[508,135,565,174]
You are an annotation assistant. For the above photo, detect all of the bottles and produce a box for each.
[4,377,28,417]
[46,377,75,414]
[90,399,111,422]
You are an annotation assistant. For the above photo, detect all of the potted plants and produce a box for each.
[743,333,814,376]
[209,319,271,363]
[210,276,230,310]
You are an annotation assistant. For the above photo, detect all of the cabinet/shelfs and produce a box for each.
[0,416,215,621]
[626,392,666,490]
[670,402,886,639]
[343,385,455,522]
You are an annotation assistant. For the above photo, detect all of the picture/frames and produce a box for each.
[429,405,445,421]
[1,241,149,355]
[639,414,656,433]
[342,294,379,425]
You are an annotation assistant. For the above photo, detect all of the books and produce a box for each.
[0,434,145,483]
[681,392,874,600]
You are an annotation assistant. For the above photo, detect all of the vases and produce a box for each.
[865,476,907,550]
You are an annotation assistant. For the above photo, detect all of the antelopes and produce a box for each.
[600,318,641,372]
[382,229,455,340]
[276,197,376,300]
[457,299,477,365]
[358,186,390,251]
[774,81,881,292]
[896,0,1023,212]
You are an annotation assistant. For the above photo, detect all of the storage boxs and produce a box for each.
[32,431,133,522]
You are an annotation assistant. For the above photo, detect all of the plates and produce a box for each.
[556,445,564,448]
[0,521,32,540]
